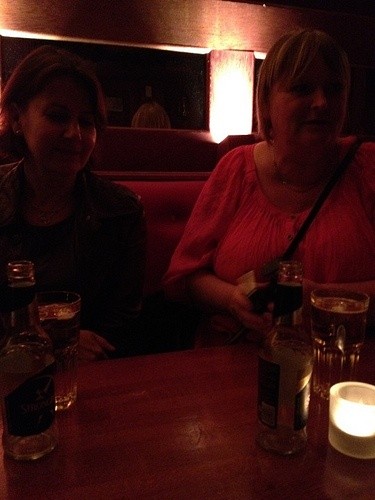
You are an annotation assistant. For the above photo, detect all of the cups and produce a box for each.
[310,287,370,401]
[36,291,81,411]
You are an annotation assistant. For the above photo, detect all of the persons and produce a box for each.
[0,45,147,365]
[156,25,375,350]
[132,100,171,130]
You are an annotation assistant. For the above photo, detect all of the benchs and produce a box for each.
[99,127,257,171]
[94,170,215,310]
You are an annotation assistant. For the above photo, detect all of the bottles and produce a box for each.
[0,260,60,463]
[257,260,314,455]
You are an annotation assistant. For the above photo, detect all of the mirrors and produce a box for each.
[0,33,211,135]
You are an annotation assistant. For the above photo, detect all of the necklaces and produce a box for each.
[271,143,313,184]
[24,203,73,225]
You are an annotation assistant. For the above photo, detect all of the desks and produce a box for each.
[0,336,375,500]
